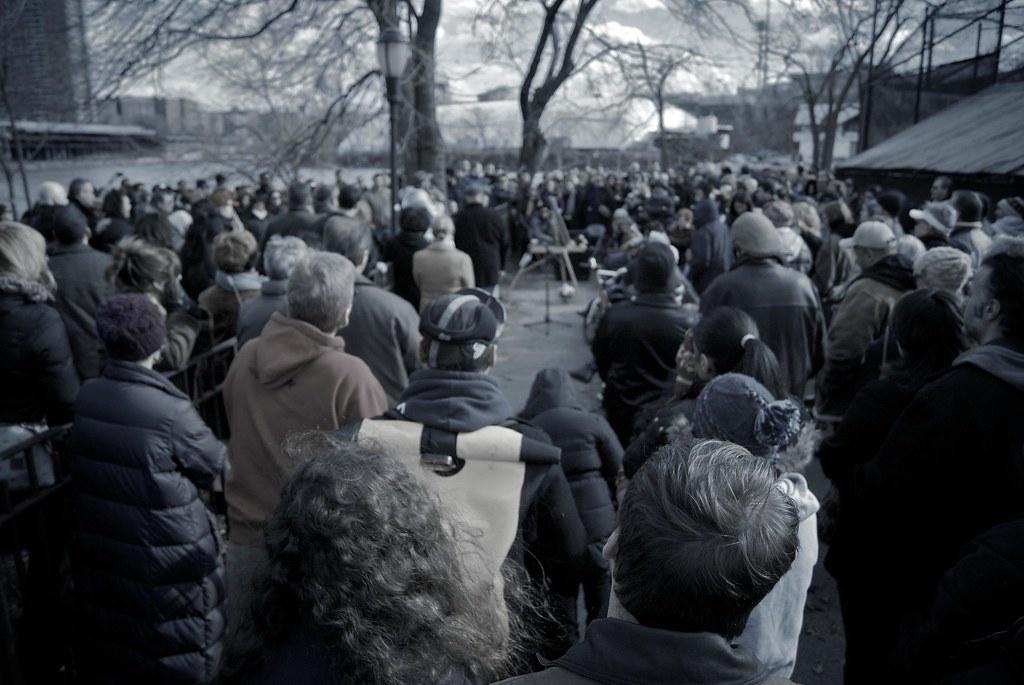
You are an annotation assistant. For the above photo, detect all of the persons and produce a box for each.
[2,121,1022,684]
[507,434,851,684]
[53,294,230,684]
[222,442,506,685]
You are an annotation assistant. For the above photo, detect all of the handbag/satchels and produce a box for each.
[0,421,56,491]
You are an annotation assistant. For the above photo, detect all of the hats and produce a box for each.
[694,373,799,459]
[838,220,897,249]
[997,196,1024,218]
[418,287,507,344]
[96,294,165,361]
[913,247,971,293]
[731,212,782,257]
[909,201,959,236]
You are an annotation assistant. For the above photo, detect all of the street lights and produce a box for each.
[376,24,411,241]
